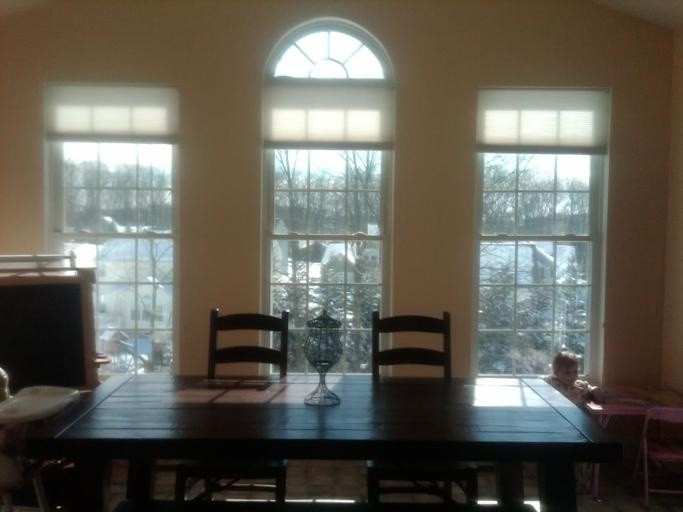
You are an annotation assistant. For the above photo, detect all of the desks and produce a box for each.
[581,385,682,501]
[23,372,623,509]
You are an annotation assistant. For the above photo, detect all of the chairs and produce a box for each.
[0,366,79,510]
[363,307,479,508]
[632,405,680,511]
[166,303,294,502]
